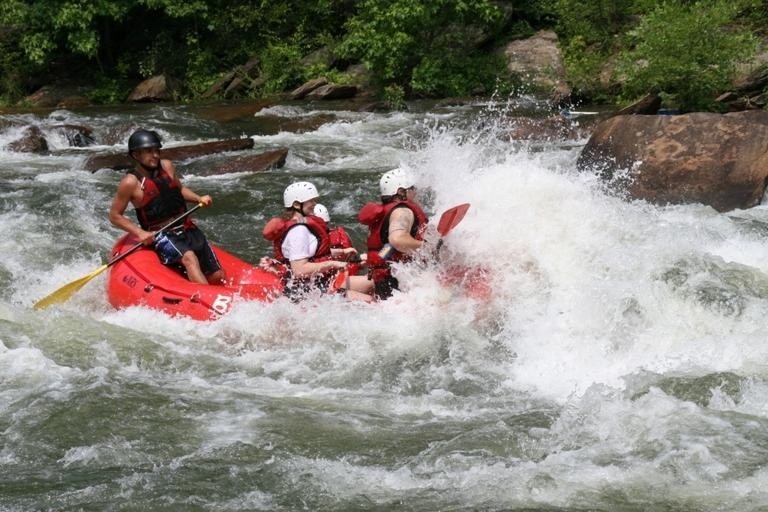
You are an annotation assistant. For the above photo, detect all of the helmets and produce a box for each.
[284,182,320,208]
[314,204,330,221]
[380,169,415,195]
[129,130,162,151]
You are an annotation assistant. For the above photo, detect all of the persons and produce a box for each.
[262,167,453,305]
[108,129,226,285]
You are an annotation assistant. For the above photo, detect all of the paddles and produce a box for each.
[332,251,352,290]
[33,202,204,311]
[433,204,470,261]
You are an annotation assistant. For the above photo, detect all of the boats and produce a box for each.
[100,231,545,338]
